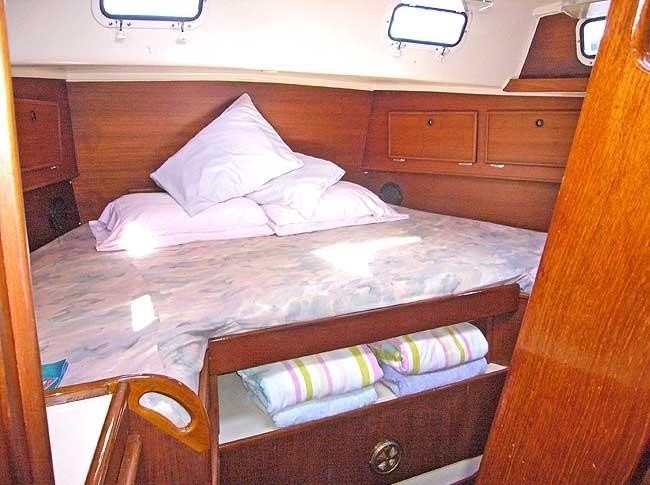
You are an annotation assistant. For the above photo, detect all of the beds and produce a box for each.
[30,207,551,485]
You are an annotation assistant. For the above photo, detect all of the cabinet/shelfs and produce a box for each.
[15,100,65,174]
[387,104,576,166]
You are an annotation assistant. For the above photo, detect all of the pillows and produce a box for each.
[244,141,346,229]
[148,92,305,219]
[98,192,265,235]
[261,180,394,226]
